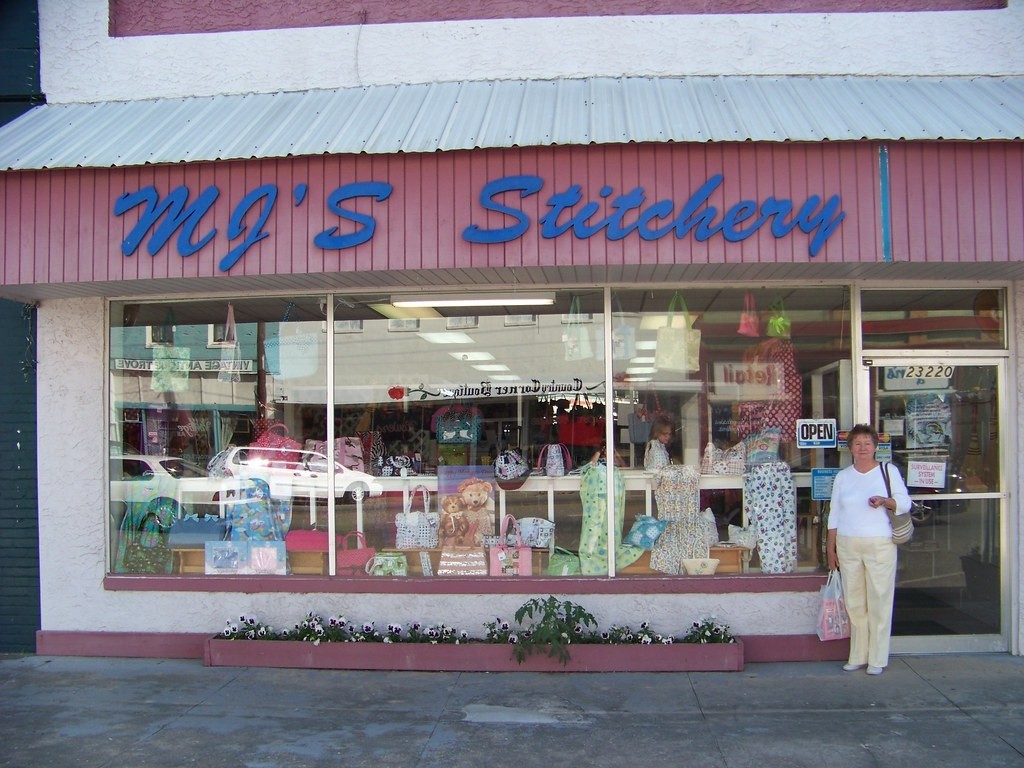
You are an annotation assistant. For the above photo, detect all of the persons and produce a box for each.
[644,416,670,469]
[827,423,911,675]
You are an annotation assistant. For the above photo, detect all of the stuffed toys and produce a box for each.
[439,479,493,547]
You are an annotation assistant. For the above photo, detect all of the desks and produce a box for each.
[178,471,812,551]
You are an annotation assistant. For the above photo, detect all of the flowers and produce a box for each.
[224,594,738,668]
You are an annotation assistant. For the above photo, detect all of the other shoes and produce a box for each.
[866,664,883,676]
[843,662,867,672]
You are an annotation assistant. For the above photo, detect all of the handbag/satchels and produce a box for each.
[124,512,179,575]
[683,538,718,574]
[309,435,364,472]
[700,441,746,475]
[700,507,720,549]
[880,460,915,545]
[488,514,533,576]
[364,552,408,577]
[167,515,226,548]
[207,445,242,477]
[728,524,757,562]
[436,394,481,445]
[155,302,320,383]
[627,390,669,444]
[558,392,604,448]
[437,520,489,576]
[336,531,375,579]
[560,289,793,373]
[537,443,572,477]
[816,566,852,641]
[287,528,343,553]
[248,423,303,469]
[494,450,528,480]
[547,545,581,576]
[395,484,440,549]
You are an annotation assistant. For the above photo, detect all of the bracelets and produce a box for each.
[880,497,887,507]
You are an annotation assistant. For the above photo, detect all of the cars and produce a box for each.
[208,445,384,505]
[723,448,971,528]
[107,454,265,532]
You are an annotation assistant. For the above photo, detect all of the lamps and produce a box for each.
[390,292,556,307]
